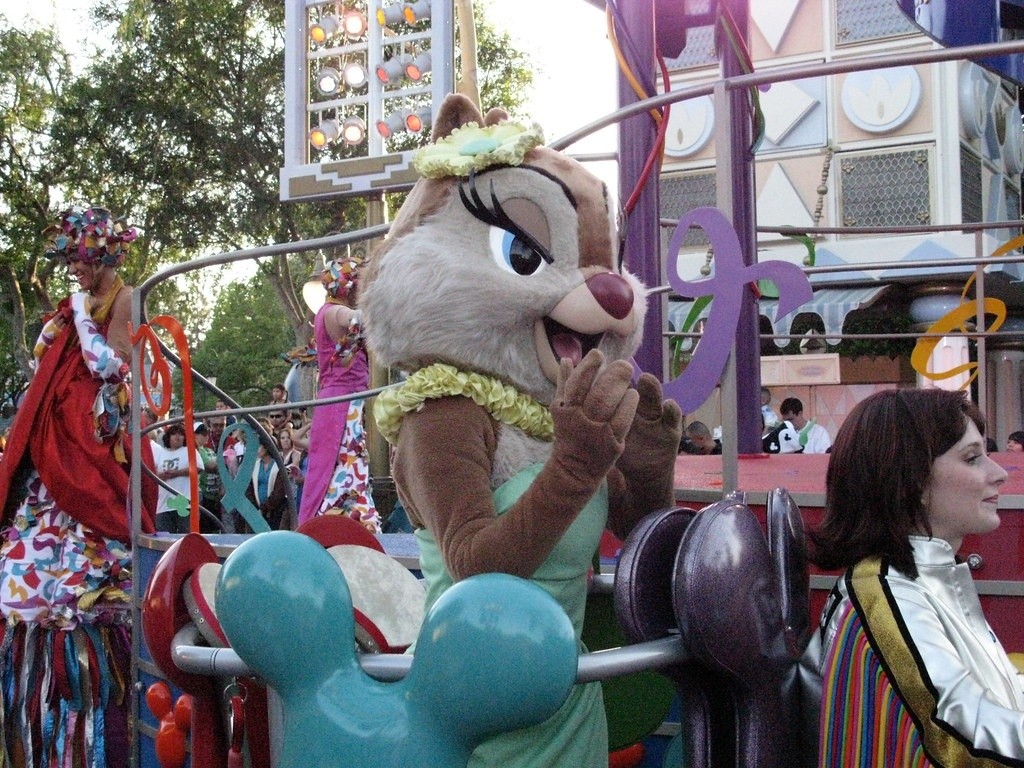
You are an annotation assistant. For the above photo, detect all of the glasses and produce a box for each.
[270,415,284,419]
[211,422,225,427]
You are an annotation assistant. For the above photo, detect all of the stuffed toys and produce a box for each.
[356,94,681,768]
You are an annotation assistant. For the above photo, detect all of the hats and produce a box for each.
[193,422,203,433]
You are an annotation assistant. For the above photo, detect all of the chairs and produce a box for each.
[614,487,803,767]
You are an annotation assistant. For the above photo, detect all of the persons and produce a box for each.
[147,385,425,580]
[803,389,1024,768]
[299,257,381,539]
[0,209,159,630]
[986,431,1024,452]
[762,385,831,454]
[684,422,722,455]
